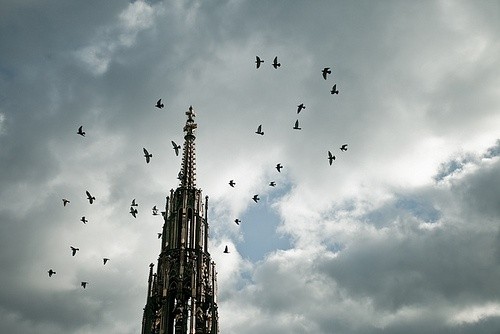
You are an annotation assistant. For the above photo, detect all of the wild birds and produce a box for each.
[48,269,56,277]
[293,119,301,130]
[327,150,336,166]
[223,245,230,253]
[252,194,260,203]
[255,55,264,68]
[330,84,339,95]
[155,99,164,109]
[70,246,79,256]
[235,219,241,225]
[229,180,235,187]
[152,210,160,215]
[269,181,276,186]
[129,207,137,218]
[103,258,110,265]
[275,164,283,172]
[254,124,264,135]
[76,125,85,136]
[296,104,306,114]
[340,144,348,151]
[86,190,95,204]
[143,147,152,163]
[80,216,88,224]
[171,141,181,157]
[81,281,88,288]
[321,67,332,80]
[62,198,70,206]
[157,233,162,239]
[272,57,280,69]
[131,198,138,206]
[152,205,158,211]
